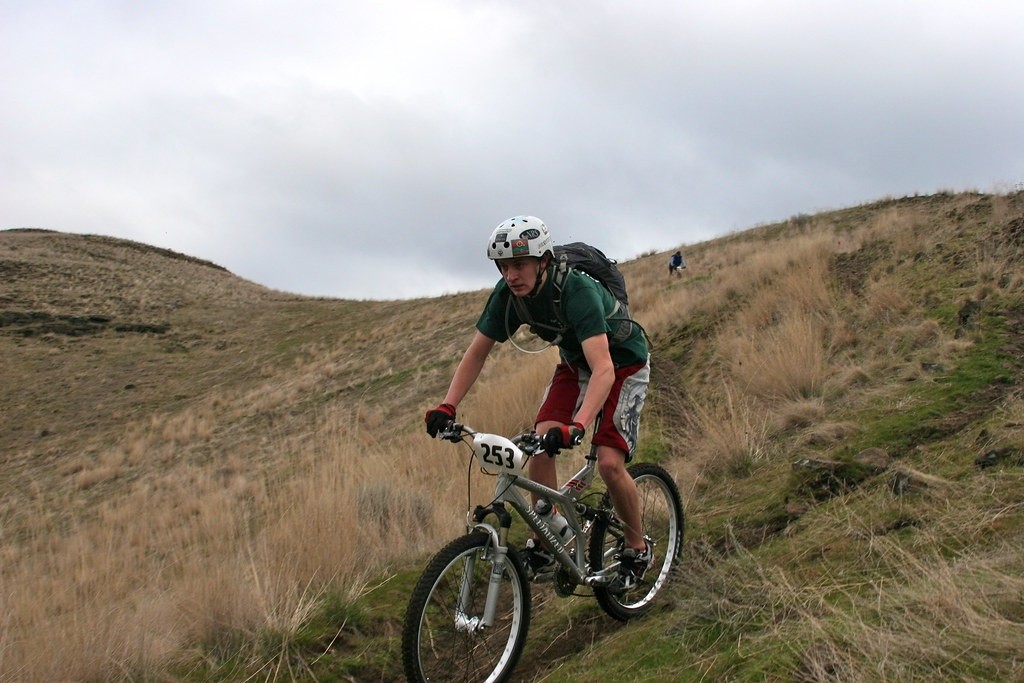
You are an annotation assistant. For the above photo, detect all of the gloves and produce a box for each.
[544,421,585,458]
[424,403,456,438]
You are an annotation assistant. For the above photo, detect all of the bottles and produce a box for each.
[534,497,575,547]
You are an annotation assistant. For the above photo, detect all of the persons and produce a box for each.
[668,250,686,276]
[424,215,654,596]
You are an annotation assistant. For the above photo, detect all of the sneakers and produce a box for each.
[607,540,656,594]
[501,538,554,580]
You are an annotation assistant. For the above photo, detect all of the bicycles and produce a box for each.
[674,266,686,280]
[400,419,686,682]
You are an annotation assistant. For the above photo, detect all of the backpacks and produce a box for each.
[551,241,628,304]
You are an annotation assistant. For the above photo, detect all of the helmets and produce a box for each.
[486,214,556,259]
